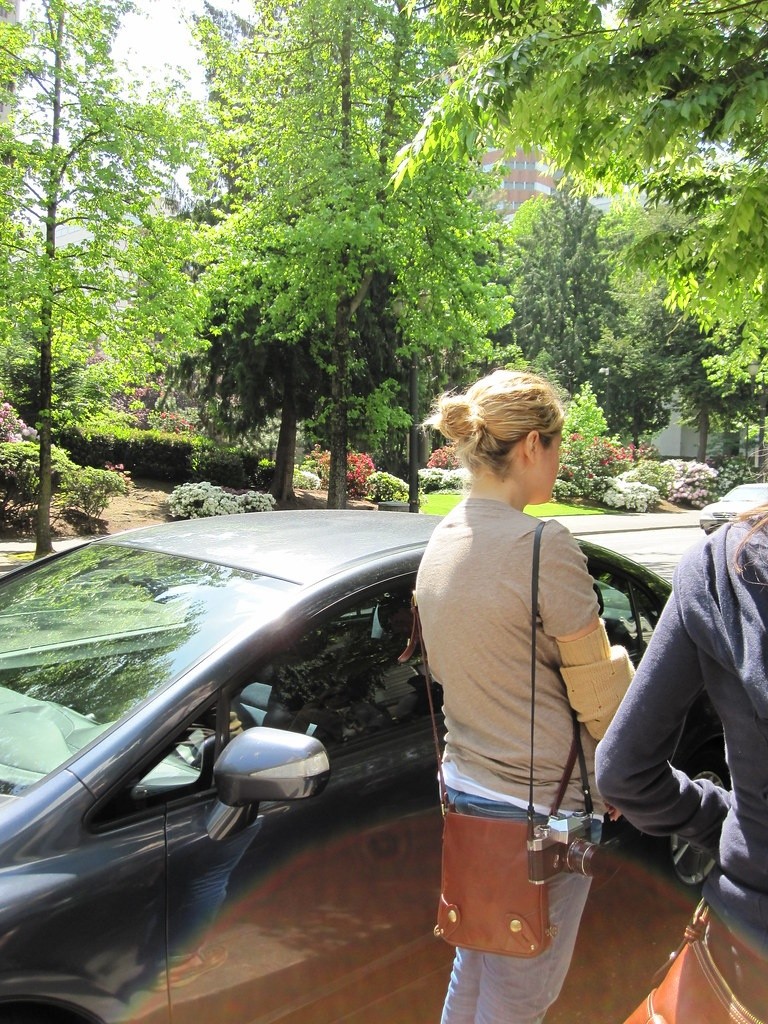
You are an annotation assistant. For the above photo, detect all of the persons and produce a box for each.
[413,367,639,1024]
[261,583,444,748]
[593,481,768,1024]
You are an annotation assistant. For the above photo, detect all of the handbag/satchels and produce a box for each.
[433,819,550,958]
[619,933,768,1024]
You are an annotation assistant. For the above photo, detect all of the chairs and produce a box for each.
[350,595,420,699]
[228,623,345,756]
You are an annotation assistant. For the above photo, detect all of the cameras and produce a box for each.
[527,809,610,885]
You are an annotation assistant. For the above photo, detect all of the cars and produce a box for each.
[1,510,734,1023]
[699,483,768,536]
